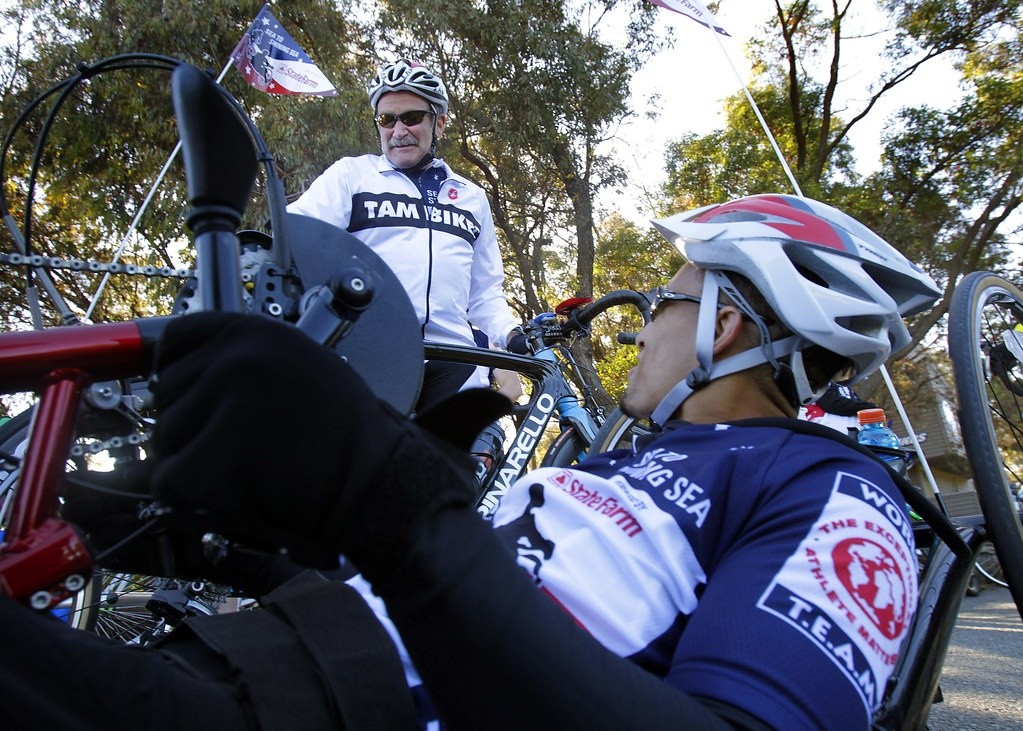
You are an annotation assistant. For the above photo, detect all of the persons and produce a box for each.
[343,193,940,731]
[285,61,530,390]
[458,317,525,406]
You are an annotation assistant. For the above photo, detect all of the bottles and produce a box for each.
[855,408,905,474]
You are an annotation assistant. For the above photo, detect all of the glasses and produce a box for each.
[650,288,754,321]
[373,110,437,129]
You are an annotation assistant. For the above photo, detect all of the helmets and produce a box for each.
[369,58,449,116]
[651,194,939,386]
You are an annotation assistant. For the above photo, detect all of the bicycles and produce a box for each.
[423,287,654,527]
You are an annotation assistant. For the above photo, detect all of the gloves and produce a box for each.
[152,310,479,582]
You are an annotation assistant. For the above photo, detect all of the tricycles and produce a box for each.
[1,51,1023,731]
